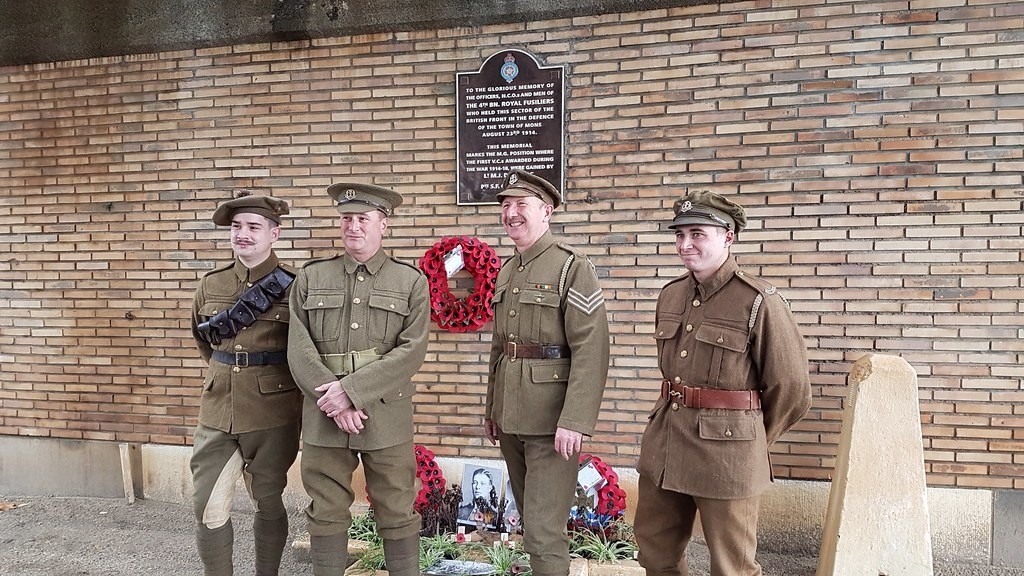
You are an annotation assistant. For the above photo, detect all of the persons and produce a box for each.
[486,164,610,576]
[188,194,302,575]
[454,469,497,526]
[287,180,432,575]
[631,191,818,576]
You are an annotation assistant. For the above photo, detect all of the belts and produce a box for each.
[659,379,762,410]
[211,350,287,369]
[320,349,388,377]
[503,340,571,360]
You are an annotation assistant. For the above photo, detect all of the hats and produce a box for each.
[496,168,562,209]
[666,190,747,235]
[211,190,291,224]
[326,182,404,217]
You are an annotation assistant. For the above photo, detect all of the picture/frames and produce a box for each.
[456,461,506,530]
